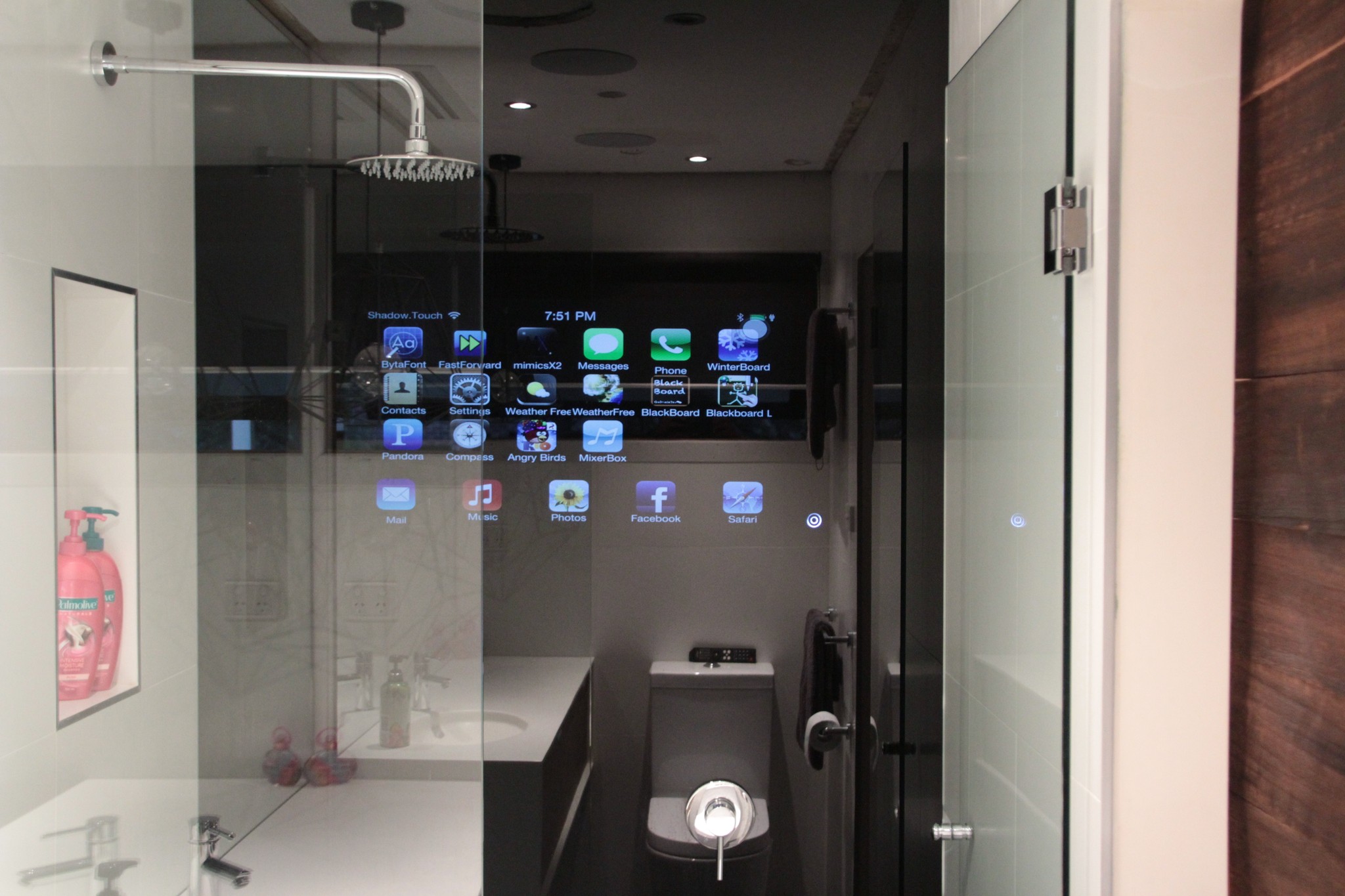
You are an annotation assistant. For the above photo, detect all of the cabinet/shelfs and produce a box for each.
[335,651,595,896]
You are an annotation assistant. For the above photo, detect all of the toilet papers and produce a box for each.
[802,712,842,770]
[870,718,879,774]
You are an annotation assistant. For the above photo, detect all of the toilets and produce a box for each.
[644,659,780,896]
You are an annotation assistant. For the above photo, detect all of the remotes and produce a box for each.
[689,647,757,664]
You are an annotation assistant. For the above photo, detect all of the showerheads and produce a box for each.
[91,42,482,182]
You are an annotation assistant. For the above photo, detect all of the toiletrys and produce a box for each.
[59,510,106,701]
[379,655,414,748]
[75,505,120,691]
[263,728,303,789]
[308,727,357,787]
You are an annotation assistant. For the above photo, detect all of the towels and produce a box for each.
[795,608,843,751]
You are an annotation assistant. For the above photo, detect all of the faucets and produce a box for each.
[402,708,531,753]
[407,652,451,707]
[16,811,136,895]
[189,817,250,896]
